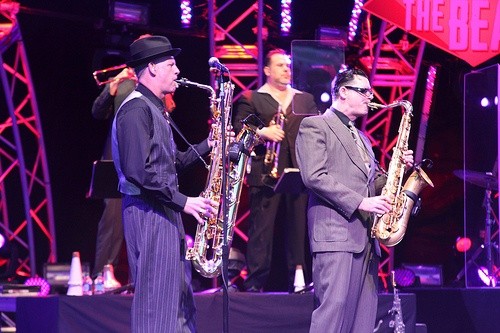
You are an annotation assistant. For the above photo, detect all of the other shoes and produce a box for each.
[103,266,122,288]
[247,286,264,293]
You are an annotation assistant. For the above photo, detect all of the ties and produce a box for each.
[348,121,370,171]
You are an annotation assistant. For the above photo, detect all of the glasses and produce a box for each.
[345,86,374,97]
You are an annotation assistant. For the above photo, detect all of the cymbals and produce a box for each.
[450,169,498,192]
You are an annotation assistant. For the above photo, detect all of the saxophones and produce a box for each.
[173,77,265,280]
[366,99,435,247]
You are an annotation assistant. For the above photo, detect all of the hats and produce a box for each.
[125,36,181,67]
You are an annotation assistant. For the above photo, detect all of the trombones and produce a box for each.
[93,64,136,86]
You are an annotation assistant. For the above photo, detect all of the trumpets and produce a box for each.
[263,103,287,180]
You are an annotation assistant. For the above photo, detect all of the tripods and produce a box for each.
[455,189,500,287]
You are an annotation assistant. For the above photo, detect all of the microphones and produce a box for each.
[208,56,230,73]
[416,159,434,170]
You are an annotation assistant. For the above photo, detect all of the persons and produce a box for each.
[112,36,236,333]
[234,49,318,294]
[92,32,176,288]
[295,68,414,333]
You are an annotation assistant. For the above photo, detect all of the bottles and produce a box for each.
[66,251,83,296]
[294,265,305,292]
[104,265,112,287]
[94,273,104,295]
[83,273,92,295]
